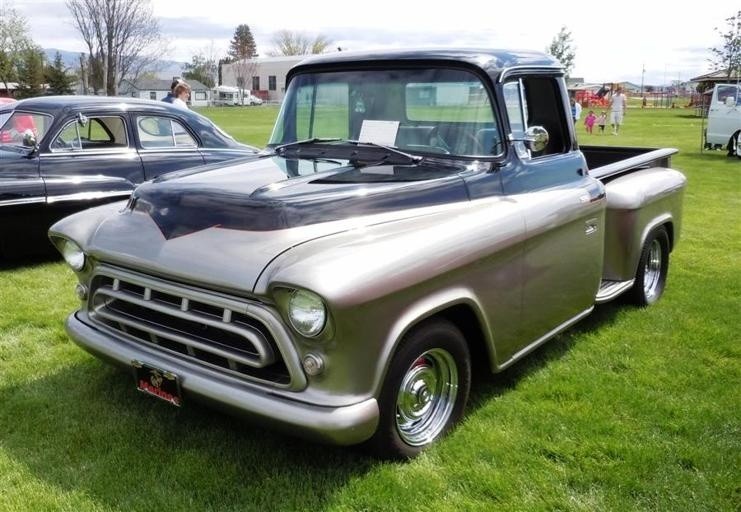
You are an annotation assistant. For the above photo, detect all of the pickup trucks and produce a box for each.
[46,44,686,465]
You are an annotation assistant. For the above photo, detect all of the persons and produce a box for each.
[606,86,627,136]
[594,110,608,134]
[156,77,185,135]
[583,110,597,134]
[169,81,192,134]
[642,95,647,107]
[569,97,583,126]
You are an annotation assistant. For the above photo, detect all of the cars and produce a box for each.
[0,95,261,266]
[705,81,741,157]
[0,96,38,145]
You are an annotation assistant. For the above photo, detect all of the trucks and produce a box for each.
[210,86,262,107]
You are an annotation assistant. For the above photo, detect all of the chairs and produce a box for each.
[473,129,524,158]
